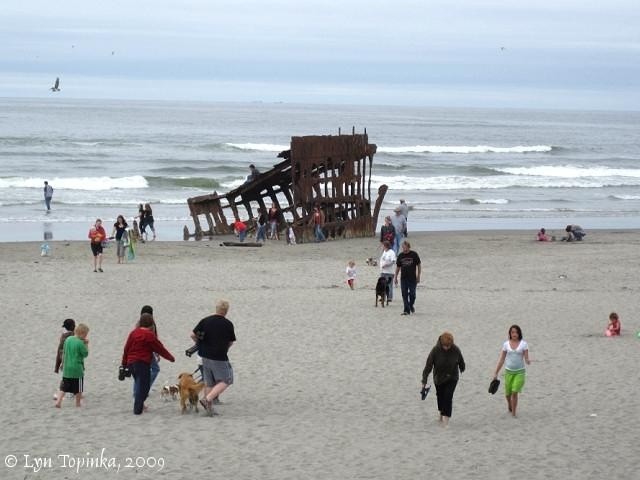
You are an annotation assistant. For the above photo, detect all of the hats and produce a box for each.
[62,319,75,331]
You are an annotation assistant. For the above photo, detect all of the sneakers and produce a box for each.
[488,379,500,394]
[199,398,214,416]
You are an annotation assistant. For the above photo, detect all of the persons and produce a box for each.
[121,313,175,415]
[89,219,106,272]
[394,241,421,314]
[110,215,129,263]
[606,312,621,336]
[537,227,555,242]
[381,216,395,253]
[54,319,84,399]
[496,325,531,417]
[392,208,407,258]
[421,331,466,424]
[308,203,327,242]
[44,181,53,211]
[379,240,397,303]
[269,202,281,240]
[140,203,156,241]
[245,164,261,183]
[191,298,236,417]
[286,221,297,245]
[231,222,246,241]
[134,204,147,241]
[255,208,266,242]
[133,305,160,409]
[566,225,586,241]
[55,324,90,407]
[398,199,409,236]
[346,260,355,290]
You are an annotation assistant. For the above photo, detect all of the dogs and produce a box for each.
[366,257,377,266]
[177,372,206,415]
[160,384,179,402]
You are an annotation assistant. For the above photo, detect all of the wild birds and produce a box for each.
[51,77,60,93]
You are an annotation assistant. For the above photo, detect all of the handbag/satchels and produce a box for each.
[101,240,111,248]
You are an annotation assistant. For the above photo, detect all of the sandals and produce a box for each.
[119,366,132,380]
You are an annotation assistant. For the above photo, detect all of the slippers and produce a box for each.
[421,385,431,400]
